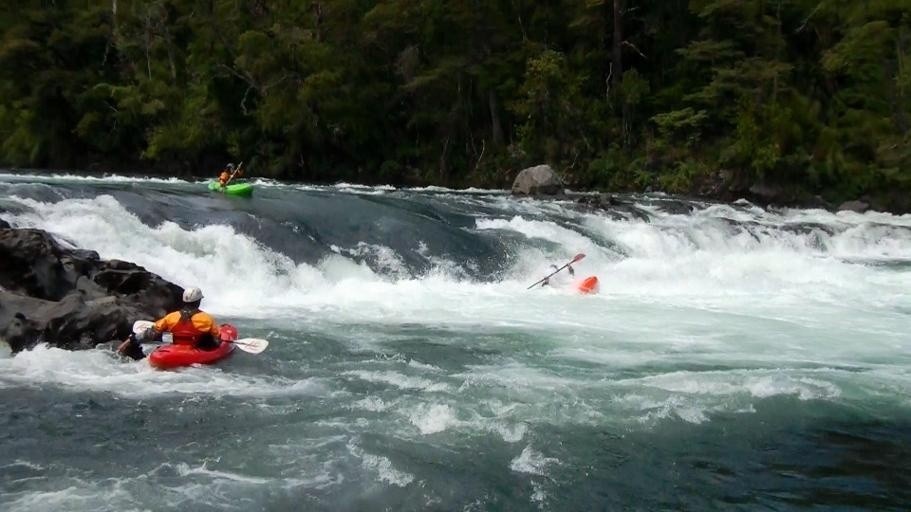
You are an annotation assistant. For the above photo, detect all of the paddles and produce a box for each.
[132,320,269,355]
[527,253,586,290]
[221,161,243,188]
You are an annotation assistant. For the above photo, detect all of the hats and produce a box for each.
[224,162,235,169]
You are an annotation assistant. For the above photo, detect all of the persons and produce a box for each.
[541,262,575,287]
[117,285,220,361]
[218,162,245,186]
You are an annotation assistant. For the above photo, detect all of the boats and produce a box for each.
[207,180,253,197]
[579,275,601,296]
[148,322,239,369]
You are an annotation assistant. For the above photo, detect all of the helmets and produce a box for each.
[183,287,204,303]
